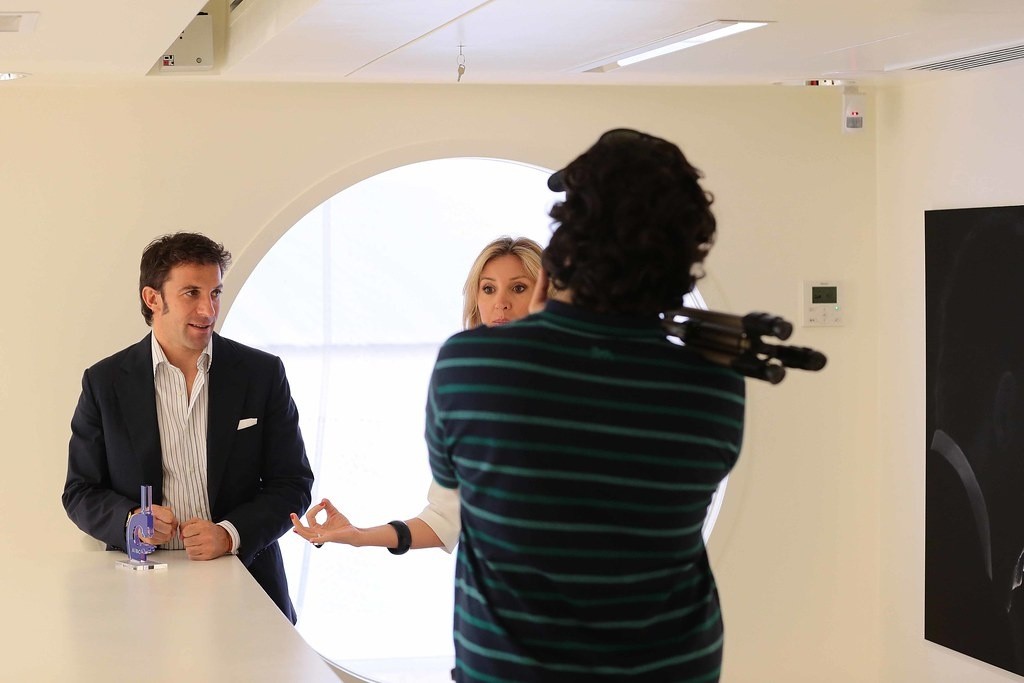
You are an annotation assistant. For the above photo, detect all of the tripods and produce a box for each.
[666,307,827,386]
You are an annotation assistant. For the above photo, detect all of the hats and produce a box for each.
[548,128,682,192]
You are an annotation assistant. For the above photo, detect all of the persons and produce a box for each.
[60,231,315,625]
[288,237,557,555]
[424,128,744,682]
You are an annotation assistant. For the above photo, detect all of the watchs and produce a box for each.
[125,508,137,531]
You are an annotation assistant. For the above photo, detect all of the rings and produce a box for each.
[312,532,324,548]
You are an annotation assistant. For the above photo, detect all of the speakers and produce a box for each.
[846,92,867,131]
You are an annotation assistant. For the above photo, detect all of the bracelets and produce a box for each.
[387,519,412,555]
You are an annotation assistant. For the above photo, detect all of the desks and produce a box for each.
[0,550,344,683]
